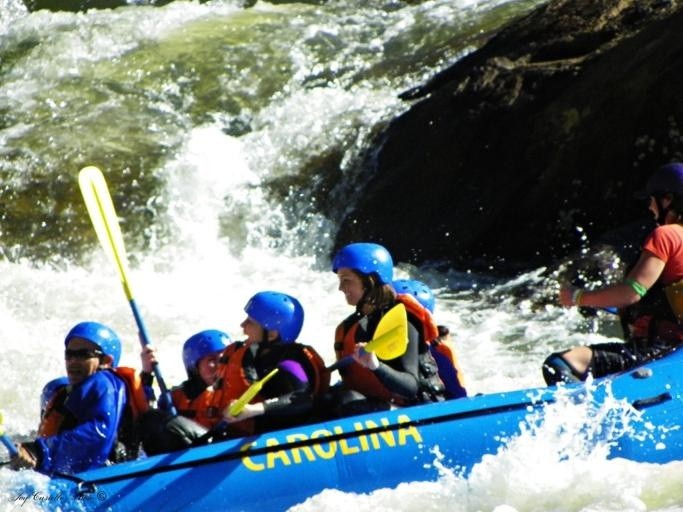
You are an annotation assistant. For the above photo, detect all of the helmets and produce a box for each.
[646,163,683,195]
[244,290,303,343]
[332,243,393,284]
[391,279,435,312]
[65,321,122,367]
[183,330,232,371]
[41,377,68,416]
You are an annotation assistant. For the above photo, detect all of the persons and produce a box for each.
[330,240,449,411]
[7,283,469,480]
[542,163,682,386]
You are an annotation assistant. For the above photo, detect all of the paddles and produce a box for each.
[329,304,408,374]
[78,166,178,420]
[210,367,279,436]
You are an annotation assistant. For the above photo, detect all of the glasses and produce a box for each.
[65,348,102,360]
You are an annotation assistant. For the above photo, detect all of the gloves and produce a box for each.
[355,342,379,371]
[224,399,264,424]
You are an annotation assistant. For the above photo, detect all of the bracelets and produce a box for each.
[568,287,588,307]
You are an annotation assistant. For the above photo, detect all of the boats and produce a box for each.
[0,346,681,512]
